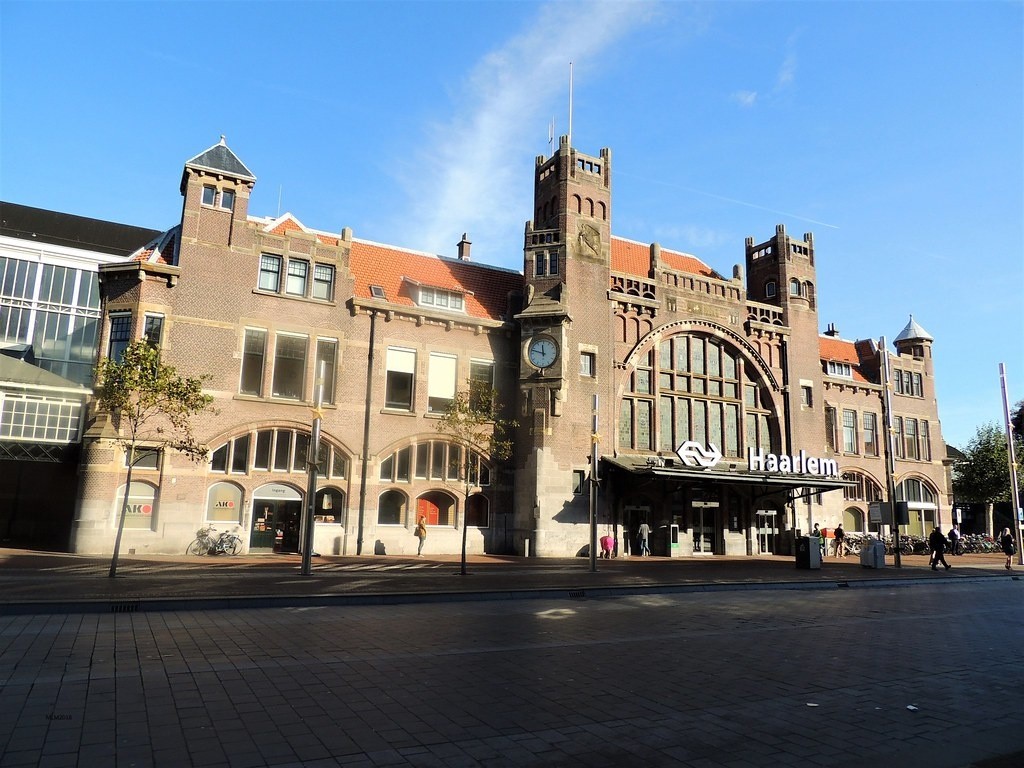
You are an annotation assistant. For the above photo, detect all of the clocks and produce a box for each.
[529,338,557,368]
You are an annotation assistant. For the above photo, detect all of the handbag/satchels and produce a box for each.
[414,527,419,536]
[1009,539,1015,555]
[819,537,823,544]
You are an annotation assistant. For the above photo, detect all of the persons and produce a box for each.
[929,529,941,565]
[833,523,848,559]
[948,523,962,556]
[600,536,614,559]
[813,523,824,561]
[931,527,952,572]
[414,514,428,555]
[1001,527,1016,571]
[635,519,653,556]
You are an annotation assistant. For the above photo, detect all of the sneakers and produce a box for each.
[418,555,424,557]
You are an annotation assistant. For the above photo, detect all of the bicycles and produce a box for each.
[826,532,1019,558]
[189,522,243,556]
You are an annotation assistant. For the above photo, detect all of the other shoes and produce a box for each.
[932,568,939,571]
[946,565,951,570]
